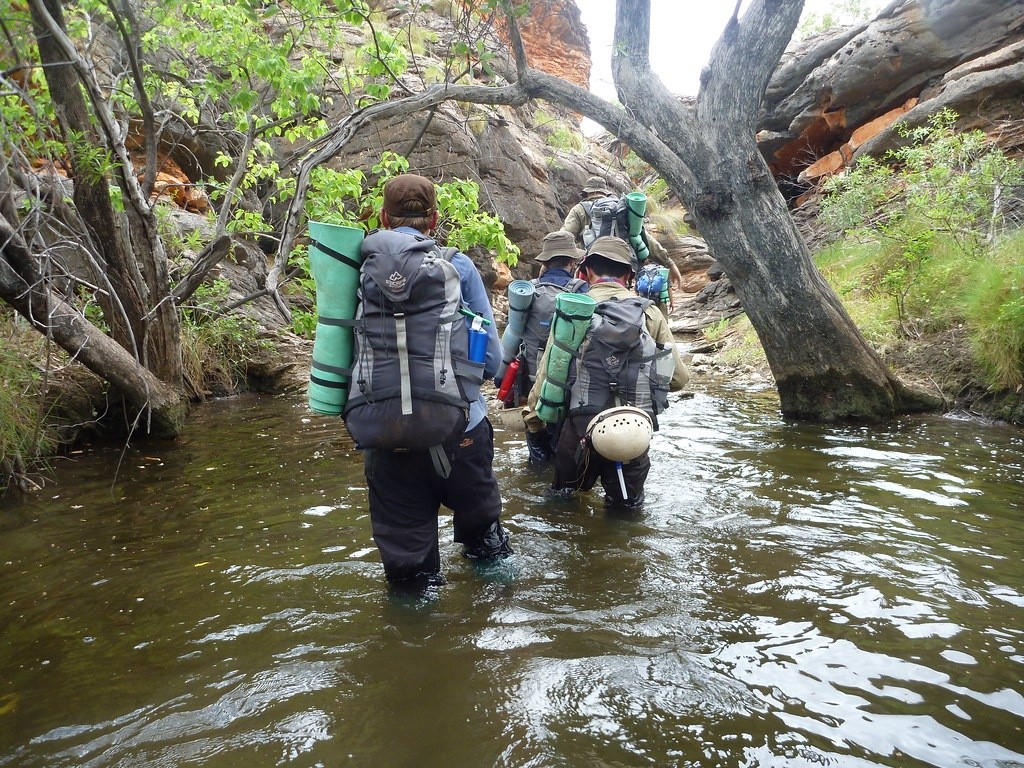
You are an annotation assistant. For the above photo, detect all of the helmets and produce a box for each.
[585,406,653,462]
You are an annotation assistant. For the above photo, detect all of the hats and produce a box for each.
[383,173,436,217]
[578,235,636,280]
[580,177,612,198]
[534,231,588,262]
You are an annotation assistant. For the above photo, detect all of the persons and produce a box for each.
[518,176,680,472]
[364,174,513,596]
[530,236,691,522]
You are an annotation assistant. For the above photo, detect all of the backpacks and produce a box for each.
[535,291,675,454]
[308,221,490,449]
[579,192,650,272]
[493,278,586,404]
[636,264,670,305]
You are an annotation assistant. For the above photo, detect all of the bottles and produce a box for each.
[468,312,489,363]
[579,269,587,281]
[583,225,595,247]
[655,343,675,385]
[496,359,520,403]
